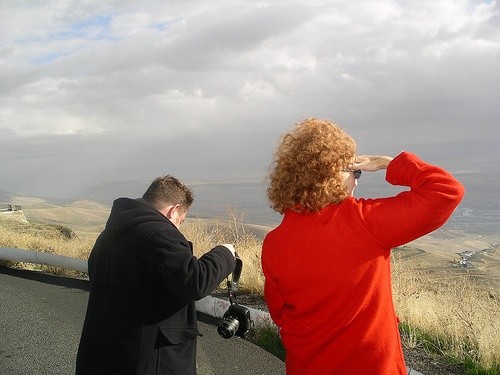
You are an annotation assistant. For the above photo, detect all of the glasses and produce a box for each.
[353,170,361,179]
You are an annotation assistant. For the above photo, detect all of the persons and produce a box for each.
[261,116,465,375]
[76,176,236,375]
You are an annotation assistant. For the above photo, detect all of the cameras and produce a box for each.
[217,305,251,339]
